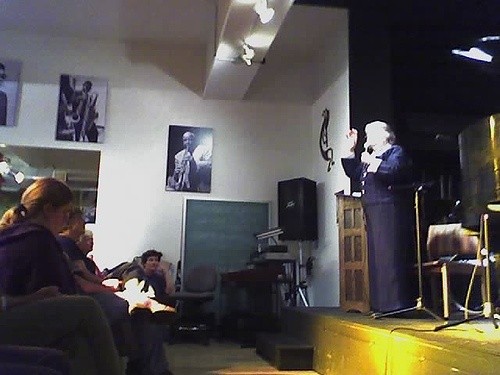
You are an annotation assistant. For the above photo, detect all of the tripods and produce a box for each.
[291,242,308,307]
[375,179,442,320]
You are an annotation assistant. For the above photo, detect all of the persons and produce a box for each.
[173,132,214,192]
[0,179,171,375]
[121,250,176,308]
[341,120,416,316]
[0,63,7,125]
[58,74,92,141]
[0,179,124,375]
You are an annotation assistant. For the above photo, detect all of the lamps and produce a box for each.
[241,41,255,66]
[250,0,276,25]
[11,170,25,184]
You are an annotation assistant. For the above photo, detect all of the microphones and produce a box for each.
[364,142,371,153]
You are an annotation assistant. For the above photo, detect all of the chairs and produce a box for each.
[168,263,217,345]
[416,213,491,320]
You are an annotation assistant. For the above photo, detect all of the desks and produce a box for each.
[251,258,298,306]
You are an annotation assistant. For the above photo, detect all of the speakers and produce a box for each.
[279,177,316,241]
